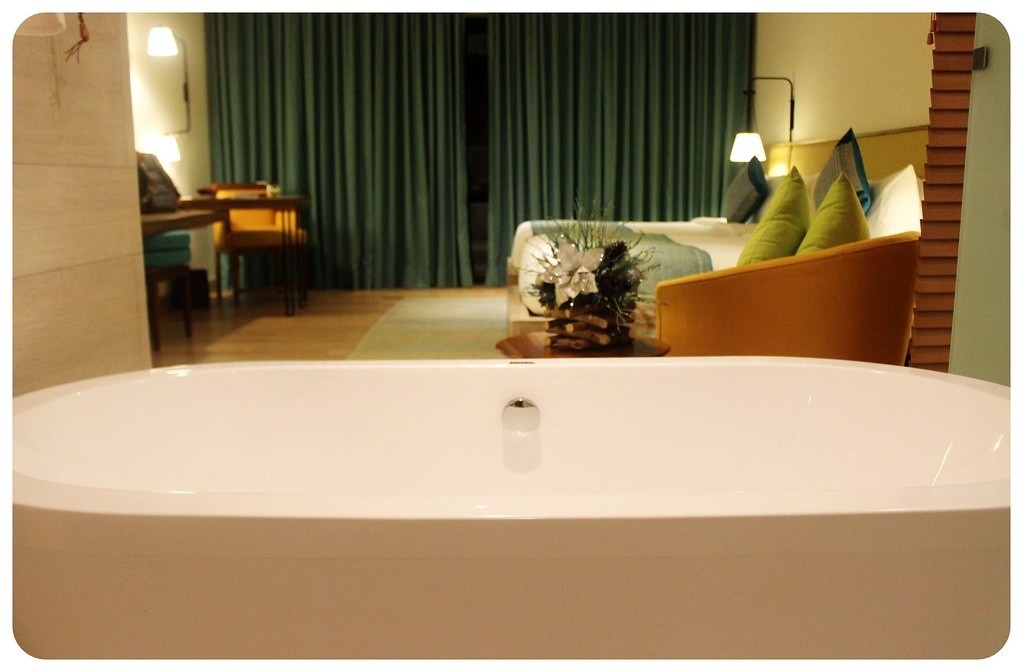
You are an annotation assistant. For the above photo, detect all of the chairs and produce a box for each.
[655,227,921,366]
[203,183,310,314]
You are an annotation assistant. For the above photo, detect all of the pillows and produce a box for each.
[814,128,871,215]
[724,156,768,223]
[737,166,810,270]
[796,172,871,254]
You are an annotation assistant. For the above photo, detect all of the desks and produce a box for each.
[499,335,670,358]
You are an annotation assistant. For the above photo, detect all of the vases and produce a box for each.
[542,308,635,348]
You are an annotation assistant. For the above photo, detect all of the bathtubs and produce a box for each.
[11,357,1011,661]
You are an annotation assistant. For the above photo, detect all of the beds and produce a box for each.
[507,124,929,335]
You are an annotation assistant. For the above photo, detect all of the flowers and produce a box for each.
[525,203,659,308]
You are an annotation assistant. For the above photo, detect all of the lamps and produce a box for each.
[731,76,795,174]
[147,27,178,58]
